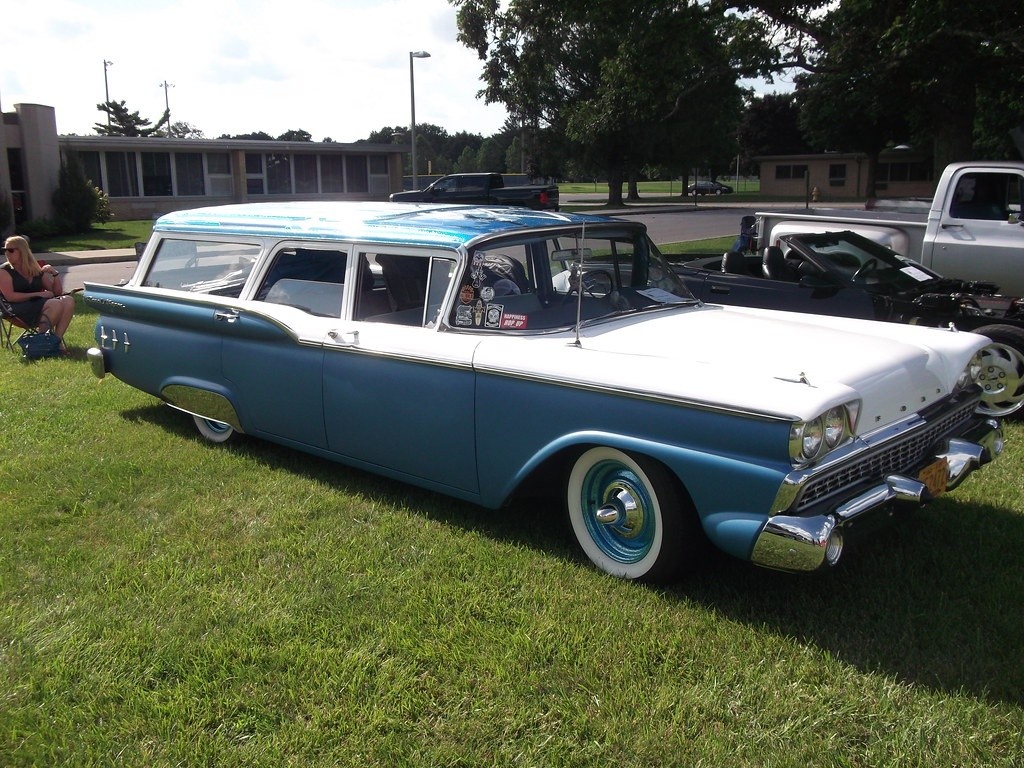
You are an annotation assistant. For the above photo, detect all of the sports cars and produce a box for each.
[571,230,1024,424]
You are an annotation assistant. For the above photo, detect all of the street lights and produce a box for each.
[409,49,431,191]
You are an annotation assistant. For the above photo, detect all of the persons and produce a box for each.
[0,235,75,356]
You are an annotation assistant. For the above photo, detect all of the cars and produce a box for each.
[78,198,1008,585]
[688,180,734,196]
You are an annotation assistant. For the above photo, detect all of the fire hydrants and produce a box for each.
[811,186,821,202]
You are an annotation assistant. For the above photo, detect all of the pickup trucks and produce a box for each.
[753,160,1024,301]
[389,173,560,211]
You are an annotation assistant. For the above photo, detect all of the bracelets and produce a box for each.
[53,273,60,276]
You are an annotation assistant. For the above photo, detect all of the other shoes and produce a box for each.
[61,349,70,355]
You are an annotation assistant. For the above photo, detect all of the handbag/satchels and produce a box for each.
[18,313,60,359]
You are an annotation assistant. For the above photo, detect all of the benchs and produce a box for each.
[362,293,542,328]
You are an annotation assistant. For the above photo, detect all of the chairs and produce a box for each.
[721,246,803,283]
[0,290,67,352]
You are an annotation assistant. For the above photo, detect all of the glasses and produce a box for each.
[1,247,17,253]
